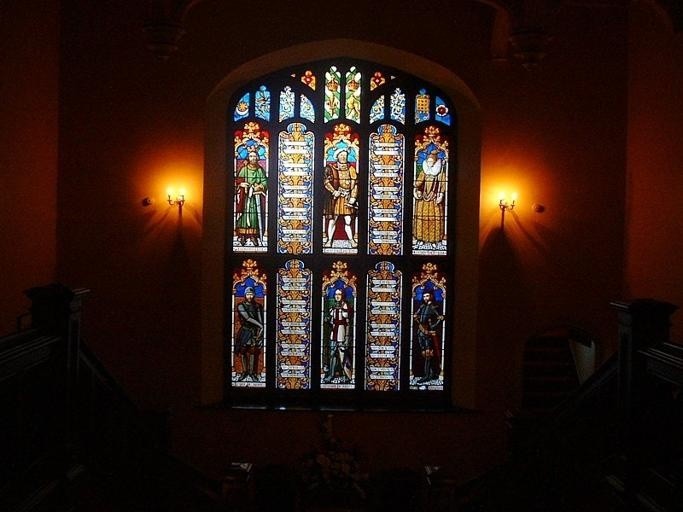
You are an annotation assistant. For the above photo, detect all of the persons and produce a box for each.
[323,287,353,384]
[234,286,263,384]
[322,147,358,249]
[413,149,448,251]
[413,289,445,384]
[233,151,267,247]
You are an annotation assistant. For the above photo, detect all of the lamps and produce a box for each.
[494,184,520,237]
[166,184,190,234]
